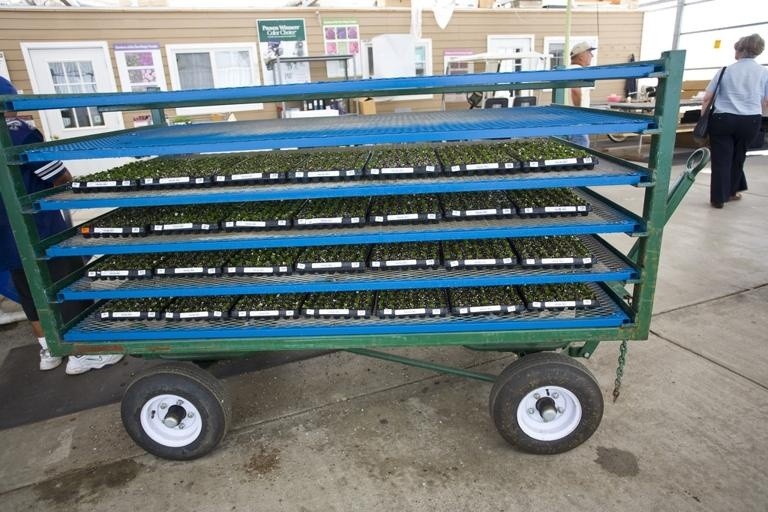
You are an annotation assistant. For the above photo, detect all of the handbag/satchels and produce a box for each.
[693,105,710,139]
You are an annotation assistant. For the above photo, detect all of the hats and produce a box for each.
[569,41,598,58]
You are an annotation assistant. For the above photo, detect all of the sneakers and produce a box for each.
[710,192,741,208]
[39,348,125,377]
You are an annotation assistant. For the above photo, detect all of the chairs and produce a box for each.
[484,96,538,109]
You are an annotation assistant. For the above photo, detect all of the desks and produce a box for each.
[614,98,704,150]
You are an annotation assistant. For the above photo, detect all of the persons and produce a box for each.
[0,75,125,375]
[566,41,598,148]
[700,32,767,208]
